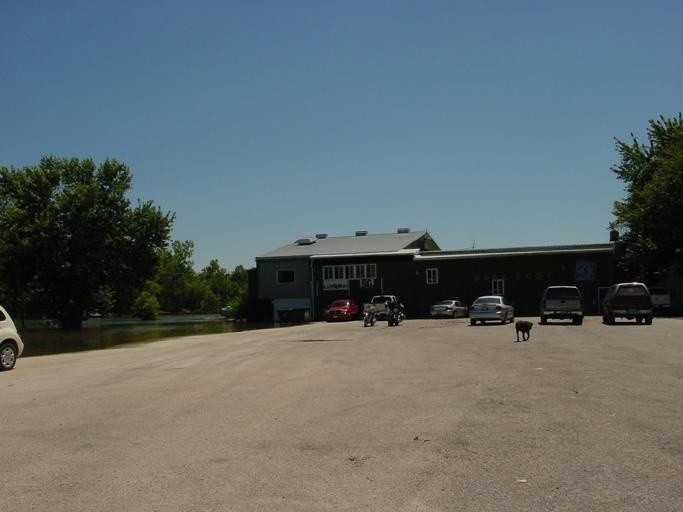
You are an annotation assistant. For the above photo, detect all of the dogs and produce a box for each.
[515,319,533,343]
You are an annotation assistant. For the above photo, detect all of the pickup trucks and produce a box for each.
[362,295,407,323]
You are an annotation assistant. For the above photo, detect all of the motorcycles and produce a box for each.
[364,297,401,327]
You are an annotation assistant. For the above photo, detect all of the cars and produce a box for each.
[602,281,672,325]
[430,296,514,324]
[0,305,24,371]
[539,285,585,324]
[323,299,359,322]
